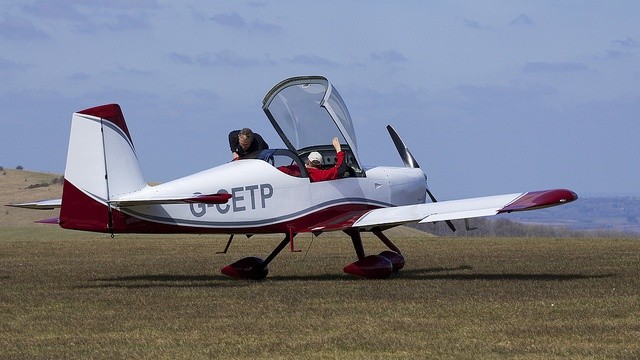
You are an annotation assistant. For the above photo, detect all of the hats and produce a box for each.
[308,152,323,165]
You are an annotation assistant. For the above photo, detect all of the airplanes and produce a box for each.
[4,74,580,283]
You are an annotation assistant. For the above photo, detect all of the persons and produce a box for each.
[276,137,345,183]
[229,128,270,163]
